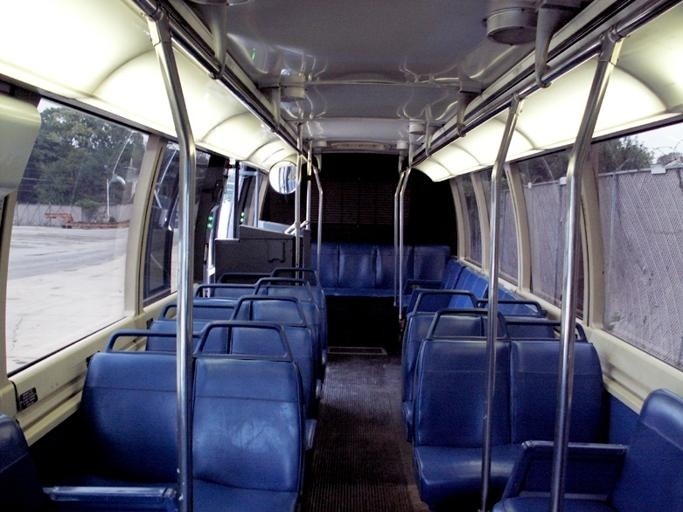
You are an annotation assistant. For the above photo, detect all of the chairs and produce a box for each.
[187,318,308,512]
[144,266,328,455]
[402,261,538,321]
[401,287,606,503]
[491,388,683,512]
[307,242,449,302]
[1,414,181,512]
[46,329,180,488]
